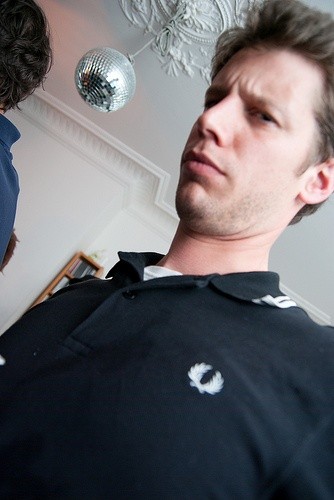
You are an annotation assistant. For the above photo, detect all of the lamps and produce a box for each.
[72,5,194,114]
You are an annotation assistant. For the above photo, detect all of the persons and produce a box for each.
[0,0,55,270]
[0,0,334,500]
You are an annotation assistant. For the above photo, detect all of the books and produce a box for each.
[39,256,97,302]
